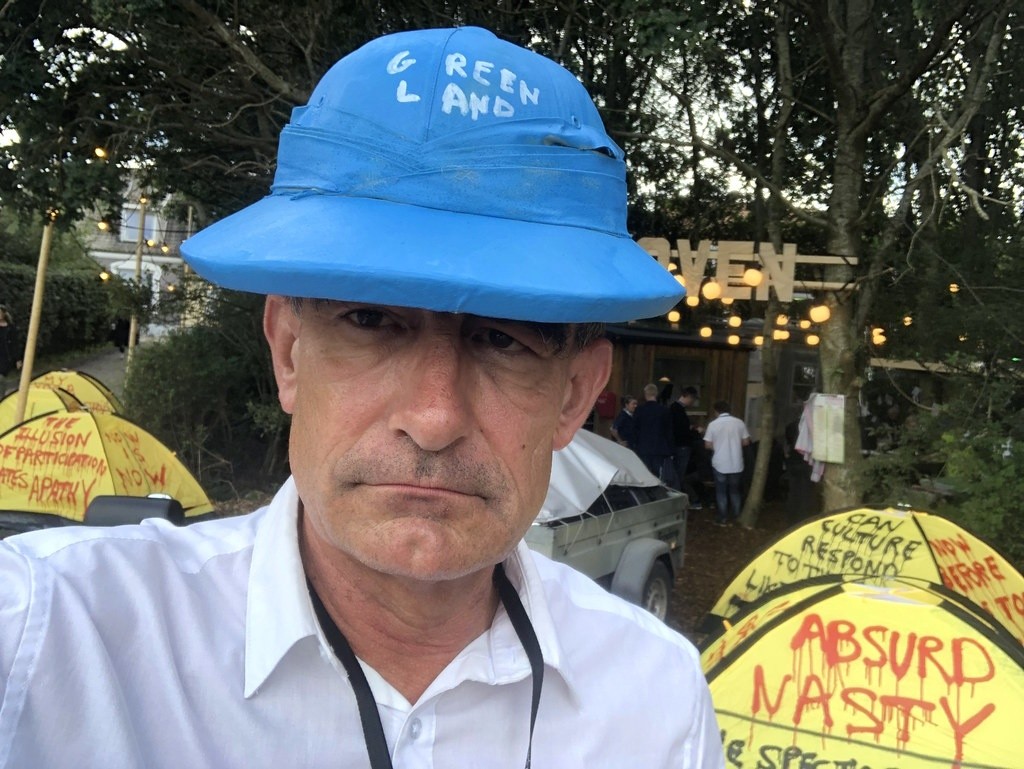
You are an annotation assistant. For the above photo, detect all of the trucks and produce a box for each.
[523,425,686,626]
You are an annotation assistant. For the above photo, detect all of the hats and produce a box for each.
[173,23,687,318]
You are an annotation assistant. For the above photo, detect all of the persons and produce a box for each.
[853,376,1024,489]
[608,393,638,453]
[106,306,140,362]
[701,400,750,528]
[655,375,677,409]
[1,25,731,769]
[626,382,675,480]
[0,303,24,401]
[659,385,704,510]
[782,398,820,517]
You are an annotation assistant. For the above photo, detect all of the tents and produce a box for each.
[0,381,86,438]
[695,572,1024,768]
[1,405,218,534]
[29,366,127,418]
[685,503,1024,647]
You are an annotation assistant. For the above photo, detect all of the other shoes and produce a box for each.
[687,498,739,527]
